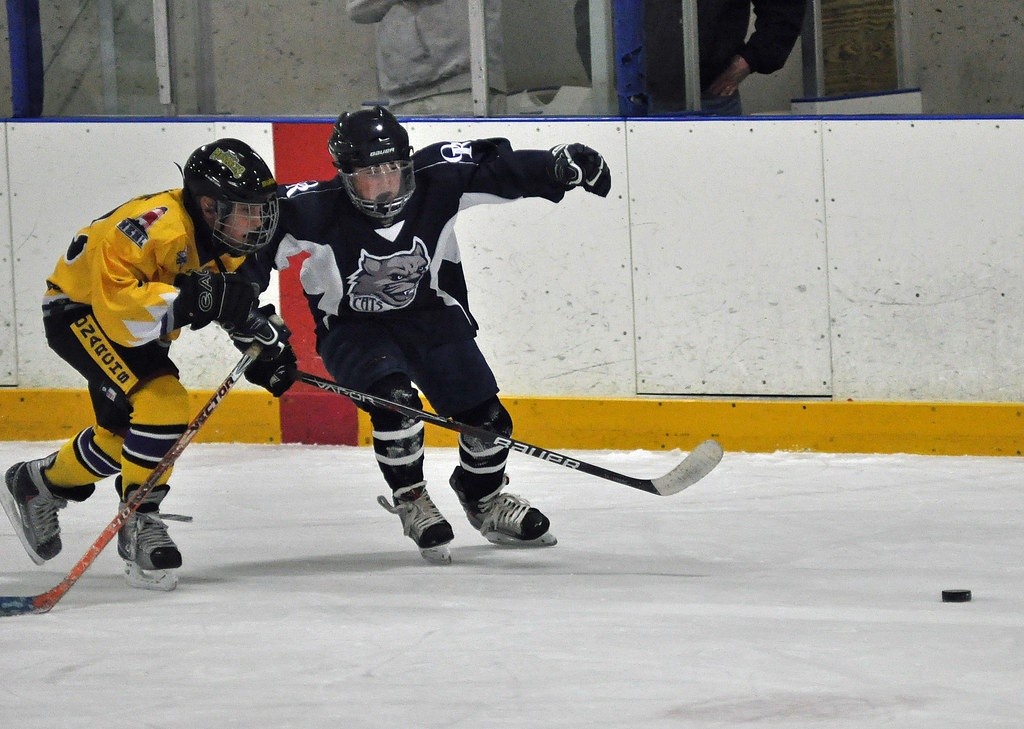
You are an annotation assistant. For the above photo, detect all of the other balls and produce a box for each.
[941,589,972,603]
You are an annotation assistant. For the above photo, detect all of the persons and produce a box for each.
[212,105,613,563]
[0,140,299,591]
[347,0,507,116]
[575,0,805,118]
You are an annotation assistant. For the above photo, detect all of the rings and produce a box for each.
[726,86,730,90]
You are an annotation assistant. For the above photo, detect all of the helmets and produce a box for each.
[329,105,417,218]
[184,137,280,257]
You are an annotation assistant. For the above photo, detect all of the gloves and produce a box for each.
[546,143,611,198]
[229,304,297,397]
[174,269,255,331]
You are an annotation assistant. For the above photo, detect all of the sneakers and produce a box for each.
[114,474,192,591]
[449,465,559,547]
[0,450,96,565]
[378,480,455,566]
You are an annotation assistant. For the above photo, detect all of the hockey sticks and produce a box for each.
[0,319,724,618]
